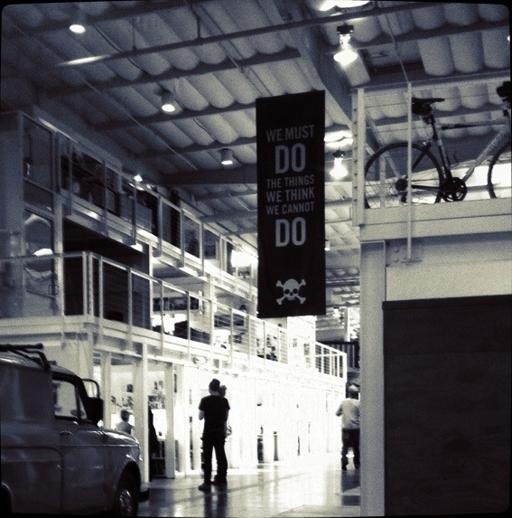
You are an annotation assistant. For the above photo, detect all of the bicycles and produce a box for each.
[363,80,512,210]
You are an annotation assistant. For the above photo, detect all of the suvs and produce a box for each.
[0,339,145,516]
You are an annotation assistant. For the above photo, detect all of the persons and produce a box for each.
[114,408,135,434]
[336,384,361,472]
[198,377,231,491]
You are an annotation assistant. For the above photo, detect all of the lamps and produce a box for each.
[333,20,359,64]
[220,149,233,166]
[161,91,176,113]
[69,8,88,34]
